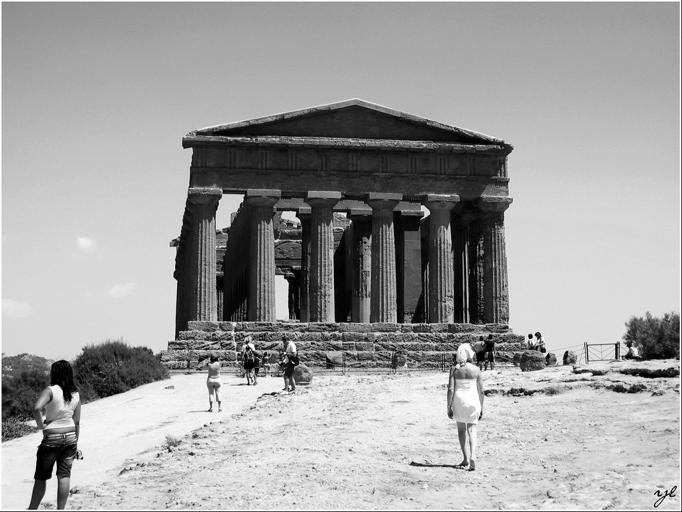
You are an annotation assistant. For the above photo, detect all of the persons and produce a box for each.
[447,344,485,470]
[625,343,639,359]
[241,335,297,392]
[28,360,81,510]
[207,353,222,413]
[472,332,546,371]
